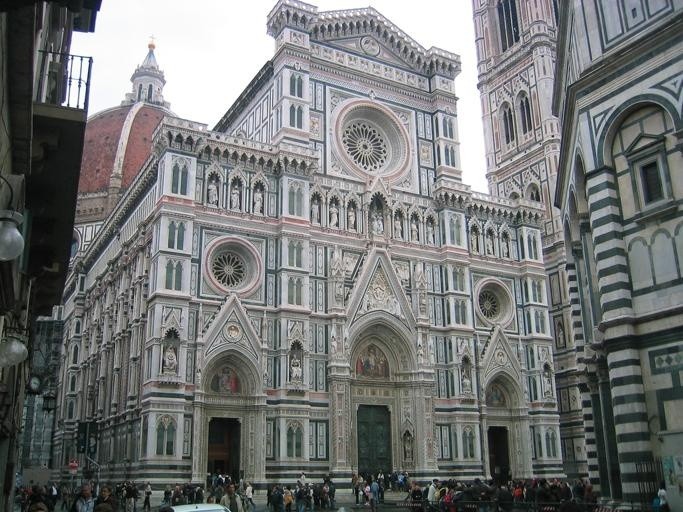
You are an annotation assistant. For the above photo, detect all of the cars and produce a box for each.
[161,503,232,512]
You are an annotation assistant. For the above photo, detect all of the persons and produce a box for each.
[253,189,263,214]
[348,207,356,228]
[329,203,338,226]
[471,231,478,252]
[394,215,402,238]
[230,186,240,210]
[652,480,667,512]
[208,180,218,205]
[290,354,302,381]
[160,473,257,512]
[427,222,434,244]
[410,220,418,241]
[486,235,493,255]
[404,477,597,512]
[15,479,152,512]
[351,470,409,512]
[267,472,336,512]
[312,199,320,224]
[502,237,508,257]
[371,213,383,234]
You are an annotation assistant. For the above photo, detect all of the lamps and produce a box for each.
[0,176,24,261]
[0,326,28,368]
